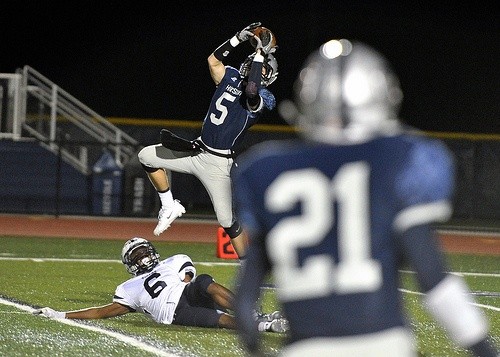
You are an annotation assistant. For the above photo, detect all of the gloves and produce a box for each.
[33,306,65,319]
[253,30,272,53]
[235,22,261,47]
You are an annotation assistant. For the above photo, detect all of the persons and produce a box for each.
[33,236,290,334]
[138,22,278,274]
[228,44,496,357]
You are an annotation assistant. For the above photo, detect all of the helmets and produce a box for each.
[121,237,160,276]
[240,52,278,87]
[297,40,403,145]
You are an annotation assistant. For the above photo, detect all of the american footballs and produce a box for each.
[250,26,276,49]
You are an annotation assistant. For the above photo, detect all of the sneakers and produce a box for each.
[255,311,291,333]
[153,199,186,236]
